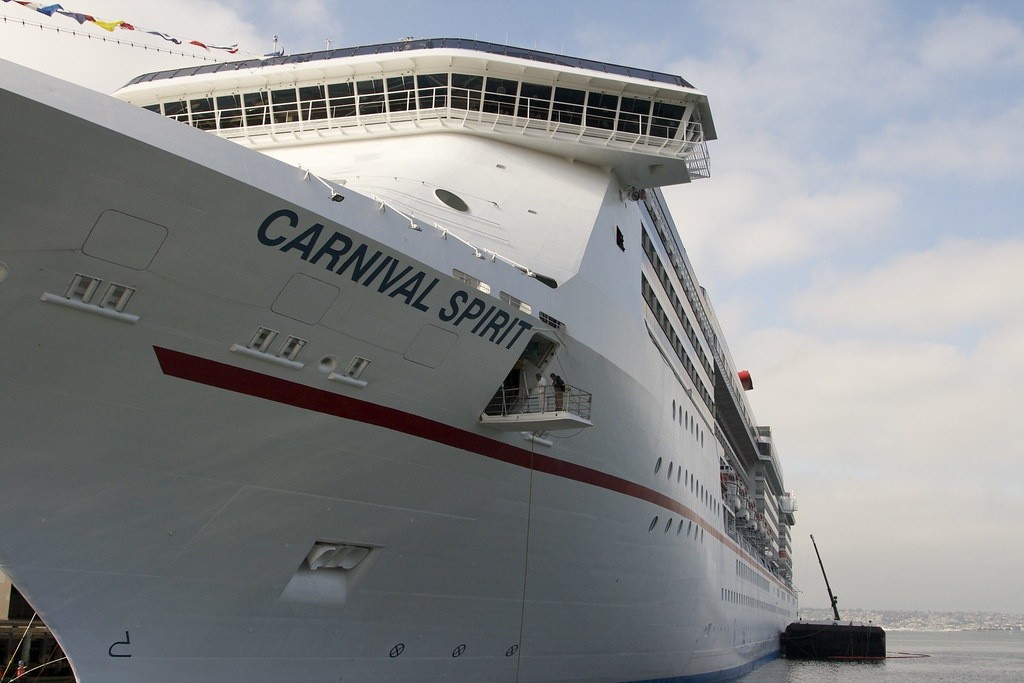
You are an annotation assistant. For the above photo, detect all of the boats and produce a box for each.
[720,469,773,547]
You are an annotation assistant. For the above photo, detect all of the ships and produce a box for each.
[1,37,796,683]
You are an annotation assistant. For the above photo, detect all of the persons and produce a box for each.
[549,373,565,410]
[534,373,546,411]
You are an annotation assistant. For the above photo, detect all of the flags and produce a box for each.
[138,27,239,53]
[3,0,134,33]
